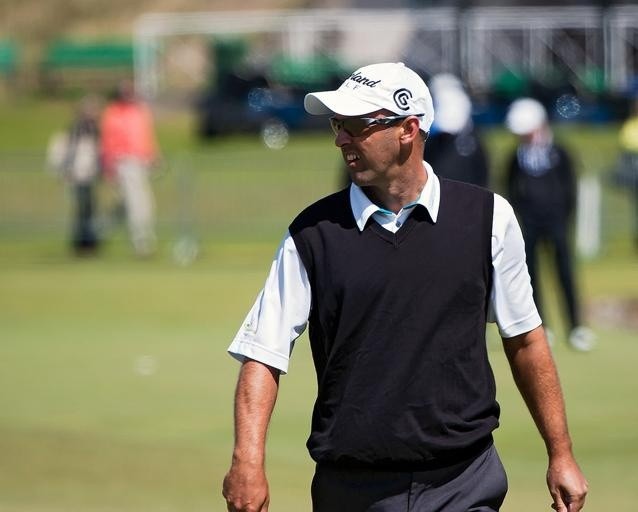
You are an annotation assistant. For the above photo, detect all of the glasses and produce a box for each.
[329,112,426,139]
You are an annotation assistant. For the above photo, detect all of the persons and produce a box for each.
[215,61,590,511]
[44,89,106,252]
[503,96,594,352]
[94,79,164,256]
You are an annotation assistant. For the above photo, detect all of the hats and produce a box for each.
[304,60,435,133]
